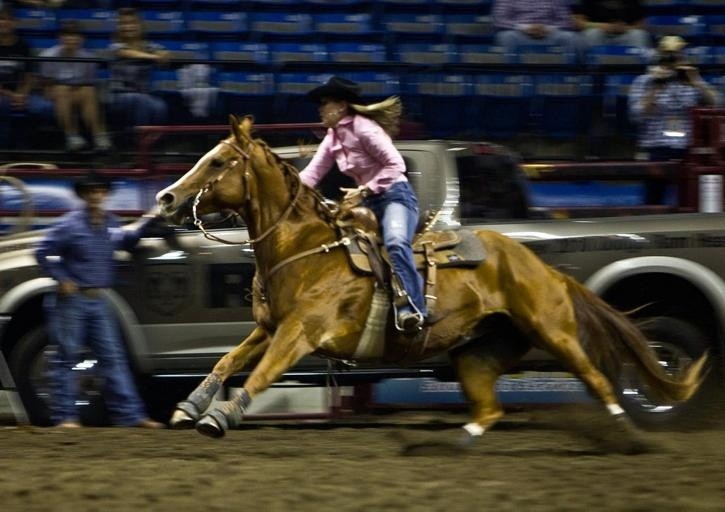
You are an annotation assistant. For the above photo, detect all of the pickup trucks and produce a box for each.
[0,130,724,436]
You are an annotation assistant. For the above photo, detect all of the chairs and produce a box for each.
[12,8,644,142]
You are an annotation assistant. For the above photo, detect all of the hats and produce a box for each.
[307,75,362,105]
[74,172,113,199]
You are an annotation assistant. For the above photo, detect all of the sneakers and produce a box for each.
[65,132,112,151]
[397,311,420,340]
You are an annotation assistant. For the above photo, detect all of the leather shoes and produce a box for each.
[53,418,166,430]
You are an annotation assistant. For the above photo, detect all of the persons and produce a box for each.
[628,34,721,205]
[294,76,429,339]
[568,1,656,56]
[0,6,52,162]
[489,1,584,62]
[100,8,175,151]
[33,19,118,157]
[31,167,168,430]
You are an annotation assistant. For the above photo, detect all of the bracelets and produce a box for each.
[358,184,369,199]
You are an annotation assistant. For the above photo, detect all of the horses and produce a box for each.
[152,112,712,459]
